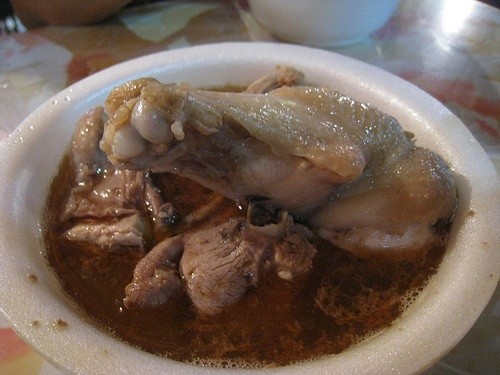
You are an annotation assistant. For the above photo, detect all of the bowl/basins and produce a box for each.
[246,0,399,49]
[0,42,500,375]
